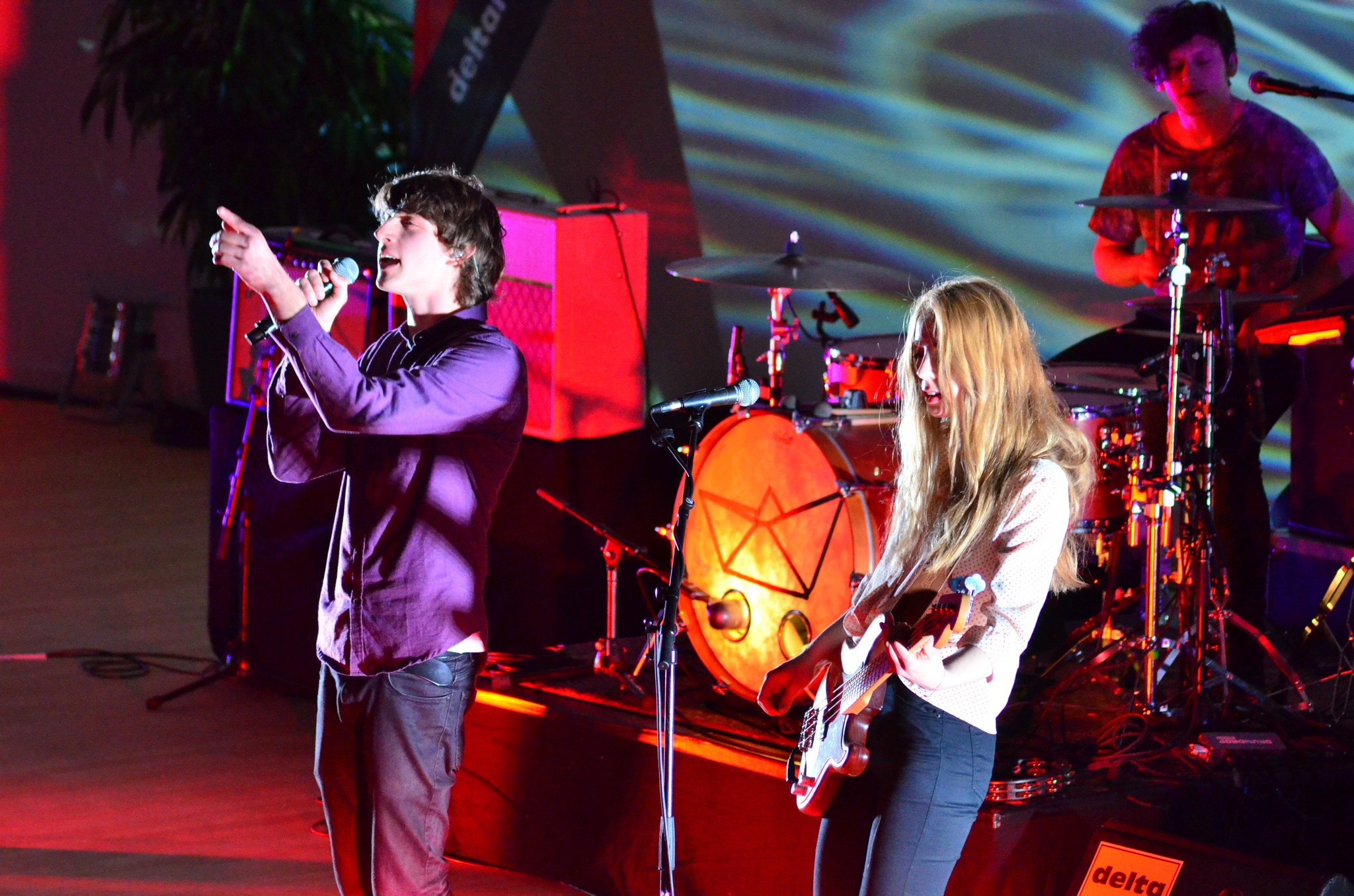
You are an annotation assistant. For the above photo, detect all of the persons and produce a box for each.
[756,275,1097,896]
[208,170,528,895]
[1038,0,1354,650]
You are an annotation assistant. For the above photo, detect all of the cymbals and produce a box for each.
[665,252,926,295]
[1123,287,1300,310]
[1074,195,1284,212]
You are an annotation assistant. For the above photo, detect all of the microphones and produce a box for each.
[649,378,761,414]
[243,258,359,347]
[1248,69,1321,99]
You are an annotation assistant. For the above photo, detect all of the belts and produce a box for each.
[402,657,453,686]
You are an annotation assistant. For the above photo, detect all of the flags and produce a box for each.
[409,0,553,182]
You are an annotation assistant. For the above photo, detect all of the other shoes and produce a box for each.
[1227,554,1271,636]
[1114,565,1168,627]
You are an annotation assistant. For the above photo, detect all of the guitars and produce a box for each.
[786,573,985,818]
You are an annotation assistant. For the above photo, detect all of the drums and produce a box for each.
[670,409,902,703]
[823,332,910,407]
[1042,362,1196,535]
